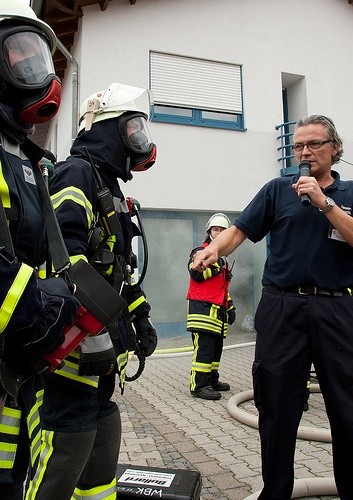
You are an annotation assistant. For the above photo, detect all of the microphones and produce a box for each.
[298,160,312,208]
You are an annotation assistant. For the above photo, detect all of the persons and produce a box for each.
[0,0,156,500]
[186,213,236,400]
[191,113,353,500]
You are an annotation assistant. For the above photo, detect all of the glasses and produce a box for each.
[293,139,331,152]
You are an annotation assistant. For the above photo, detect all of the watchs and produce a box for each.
[319,197,336,213]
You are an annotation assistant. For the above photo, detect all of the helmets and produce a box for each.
[77,86,149,133]
[206,214,229,233]
[0,0,57,59]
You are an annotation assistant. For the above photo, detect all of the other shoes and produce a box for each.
[191,386,221,399]
[212,382,230,391]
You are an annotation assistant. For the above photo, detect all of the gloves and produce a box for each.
[134,315,158,357]
[227,301,237,324]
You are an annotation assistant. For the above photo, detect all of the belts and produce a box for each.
[262,285,353,297]
